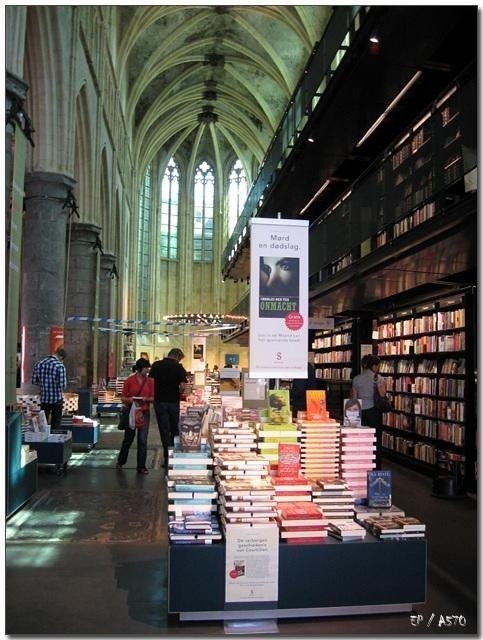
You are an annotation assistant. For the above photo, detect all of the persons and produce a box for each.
[154,356,159,361]
[116,357,155,474]
[31,348,68,430]
[149,348,188,467]
[140,352,153,376]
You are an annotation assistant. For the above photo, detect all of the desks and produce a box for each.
[167,526,428,621]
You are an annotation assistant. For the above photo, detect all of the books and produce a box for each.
[132,397,143,400]
[98,379,125,403]
[163,106,466,545]
[21,408,98,468]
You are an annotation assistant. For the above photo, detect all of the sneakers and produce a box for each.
[137,467,147,474]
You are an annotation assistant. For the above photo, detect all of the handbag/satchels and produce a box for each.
[117,406,128,430]
[372,373,392,413]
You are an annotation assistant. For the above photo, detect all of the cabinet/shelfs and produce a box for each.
[309,94,477,495]
